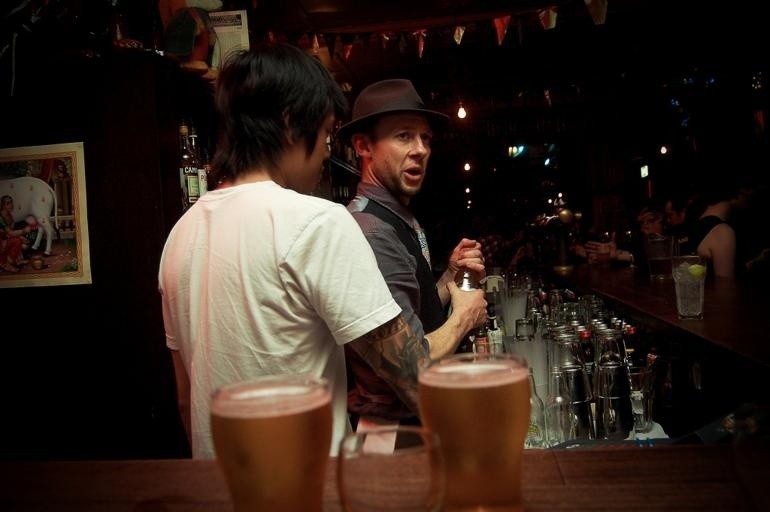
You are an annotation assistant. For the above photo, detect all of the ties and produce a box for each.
[411,217,433,275]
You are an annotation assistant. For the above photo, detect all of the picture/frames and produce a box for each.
[0,141,92,289]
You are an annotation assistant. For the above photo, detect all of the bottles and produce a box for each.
[179,121,213,208]
[572,311,646,401]
[524,366,578,450]
[505,266,578,313]
[445,267,505,360]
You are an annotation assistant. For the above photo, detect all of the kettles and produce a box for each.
[331,184,351,208]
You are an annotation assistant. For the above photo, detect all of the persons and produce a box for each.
[0,195,38,272]
[511,165,769,278]
[336,79,486,449]
[159,7,220,80]
[158,44,434,459]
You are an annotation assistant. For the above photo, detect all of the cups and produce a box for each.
[630,366,655,433]
[208,373,334,512]
[645,235,680,282]
[671,255,708,321]
[336,426,447,511]
[417,355,529,512]
[513,294,606,342]
[557,328,632,442]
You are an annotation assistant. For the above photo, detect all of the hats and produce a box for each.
[334,75,458,149]
